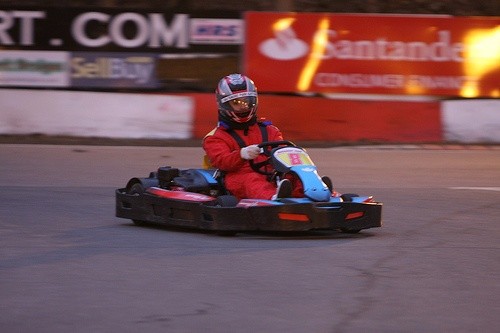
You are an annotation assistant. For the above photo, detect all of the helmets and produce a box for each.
[216,73,258,104]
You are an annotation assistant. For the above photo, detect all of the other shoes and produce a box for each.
[321,175,332,195]
[276,179,292,197]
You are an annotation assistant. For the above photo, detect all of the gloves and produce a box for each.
[240,144,260,159]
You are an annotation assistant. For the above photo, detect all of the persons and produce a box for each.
[200,72,334,204]
